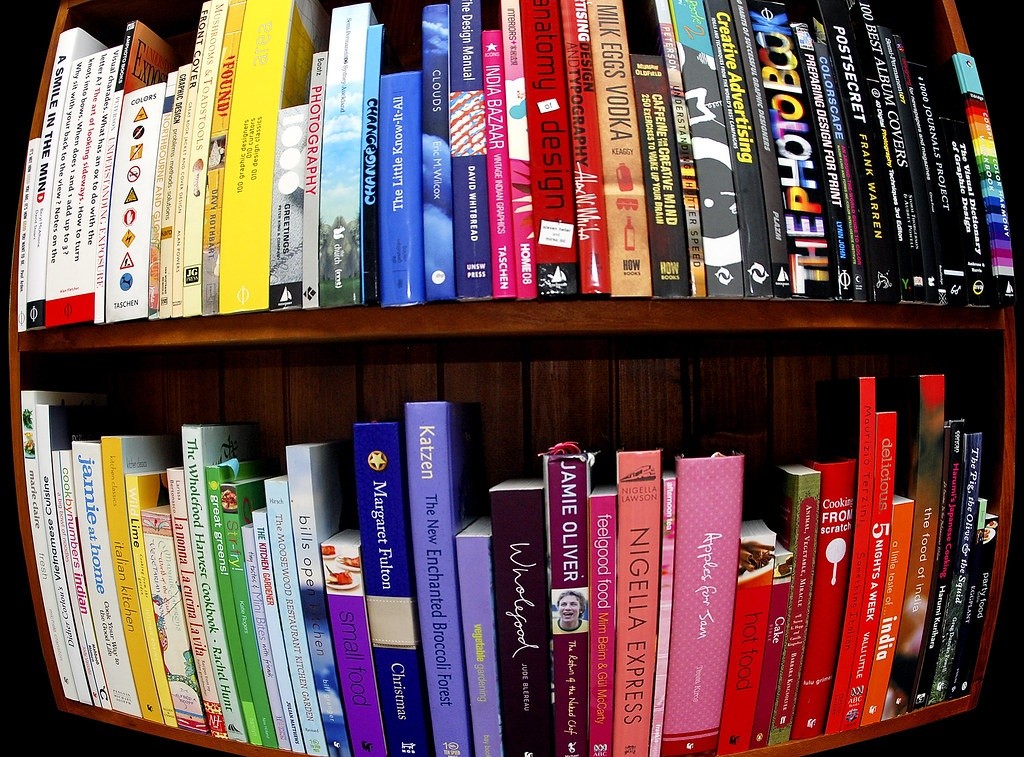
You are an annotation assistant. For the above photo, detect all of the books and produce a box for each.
[14,1,1024,334]
[22,374,1000,756]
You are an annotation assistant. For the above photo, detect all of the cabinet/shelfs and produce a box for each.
[7,0,1018,757]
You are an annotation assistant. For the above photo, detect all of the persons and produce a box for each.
[551,589,589,634]
